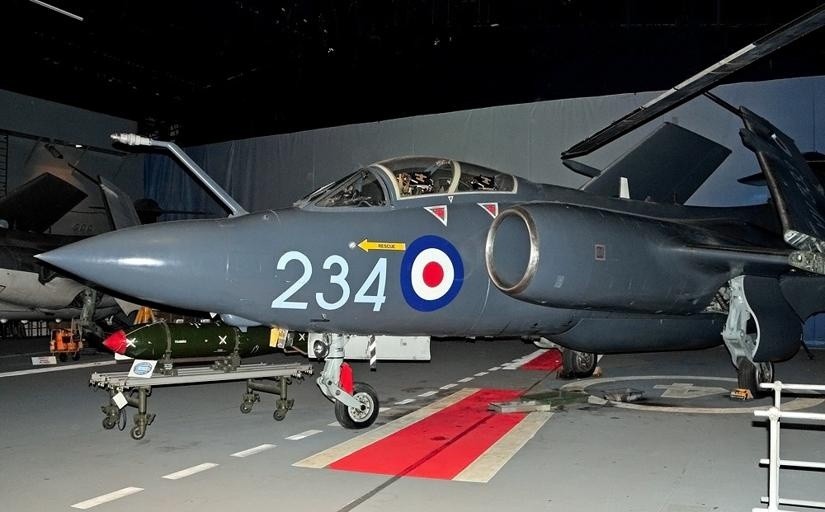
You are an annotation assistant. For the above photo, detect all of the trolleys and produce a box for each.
[88,362,313,440]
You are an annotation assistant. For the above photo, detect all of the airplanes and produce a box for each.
[1,171,147,363]
[31,0,825,430]
